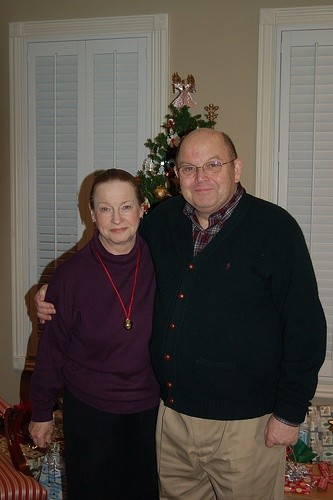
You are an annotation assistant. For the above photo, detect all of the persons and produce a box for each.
[28,168,161,500]
[35,125,329,500]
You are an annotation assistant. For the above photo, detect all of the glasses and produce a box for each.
[177,159,235,179]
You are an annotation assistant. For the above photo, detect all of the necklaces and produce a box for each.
[91,241,144,330]
